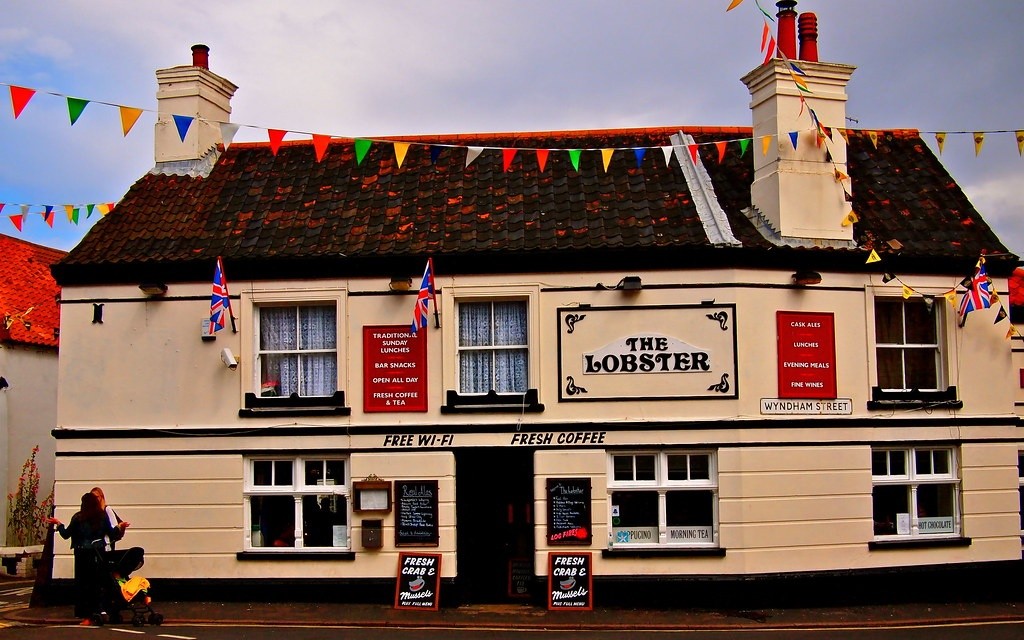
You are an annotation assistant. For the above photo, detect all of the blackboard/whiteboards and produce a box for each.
[394,479,440,548]
[392,551,443,611]
[546,477,593,547]
[545,550,594,613]
[506,556,534,599]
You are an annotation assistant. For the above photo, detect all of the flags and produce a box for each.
[958,262,990,316]
[209,257,228,336]
[410,259,434,335]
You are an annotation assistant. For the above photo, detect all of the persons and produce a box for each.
[91,487,119,550]
[46,493,130,626]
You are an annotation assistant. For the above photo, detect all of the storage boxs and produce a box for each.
[317,479,336,486]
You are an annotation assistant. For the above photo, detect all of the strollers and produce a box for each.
[91,539,163,627]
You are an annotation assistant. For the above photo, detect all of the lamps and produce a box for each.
[139,280,169,297]
[389,275,413,291]
[623,276,642,294]
[791,269,822,285]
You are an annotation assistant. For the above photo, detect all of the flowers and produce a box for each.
[6,443,53,546]
